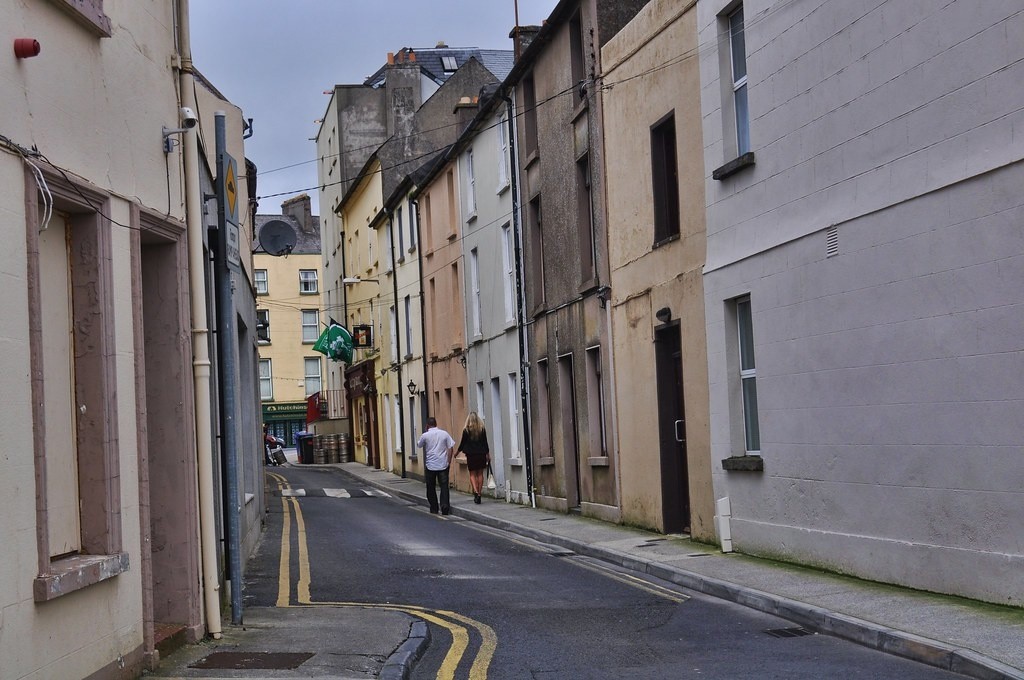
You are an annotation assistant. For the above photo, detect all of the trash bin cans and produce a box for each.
[294,431,313,464]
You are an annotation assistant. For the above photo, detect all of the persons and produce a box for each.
[454,411,491,504]
[418,417,455,515]
[263,424,270,465]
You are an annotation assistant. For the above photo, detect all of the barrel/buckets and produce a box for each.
[273,450,287,466]
[313,433,350,464]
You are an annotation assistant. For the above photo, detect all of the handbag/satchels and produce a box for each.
[486,461,497,489]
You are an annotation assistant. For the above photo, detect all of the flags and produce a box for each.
[306,391,321,423]
[311,315,353,361]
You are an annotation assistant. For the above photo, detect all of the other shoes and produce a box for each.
[442,511,448,515]
[474,493,482,504]
[430,510,437,514]
